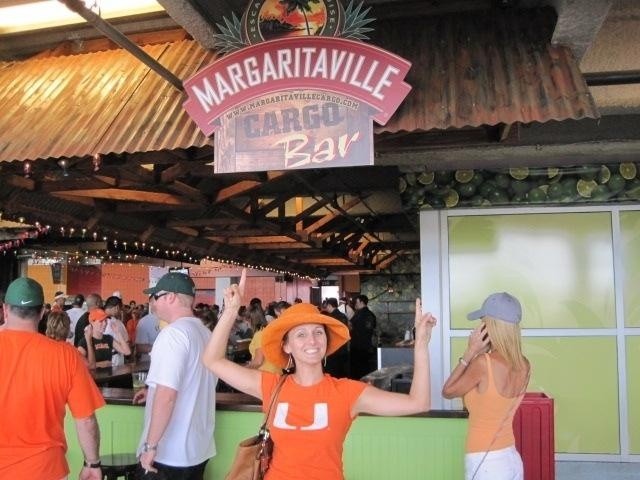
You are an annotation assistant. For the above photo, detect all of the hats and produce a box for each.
[143,272,196,297]
[261,302,351,368]
[467,292,522,325]
[89,308,112,322]
[5,276,44,307]
[55,293,69,299]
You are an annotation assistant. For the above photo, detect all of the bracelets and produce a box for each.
[84,460,102,468]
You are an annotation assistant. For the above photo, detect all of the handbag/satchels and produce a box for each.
[225,372,287,480]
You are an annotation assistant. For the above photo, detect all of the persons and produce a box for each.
[45,310,96,370]
[136,304,145,317]
[202,310,219,332]
[293,298,303,304]
[264,301,277,324]
[247,298,262,311]
[229,305,251,346]
[38,303,51,335]
[78,308,132,370]
[133,272,219,479]
[442,292,531,480]
[1,277,106,480]
[326,298,348,376]
[135,294,160,361]
[52,291,69,312]
[193,302,203,317]
[102,296,129,366]
[203,268,437,480]
[203,303,212,311]
[320,298,328,312]
[212,304,219,316]
[243,303,284,376]
[126,308,141,346]
[74,292,103,348]
[126,309,142,351]
[348,294,376,359]
[129,300,136,309]
[271,300,290,319]
[64,294,87,346]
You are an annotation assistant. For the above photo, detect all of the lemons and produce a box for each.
[399,162,640,210]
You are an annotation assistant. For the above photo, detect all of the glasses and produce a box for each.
[153,293,169,301]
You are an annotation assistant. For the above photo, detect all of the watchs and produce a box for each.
[141,442,158,453]
[459,357,469,367]
